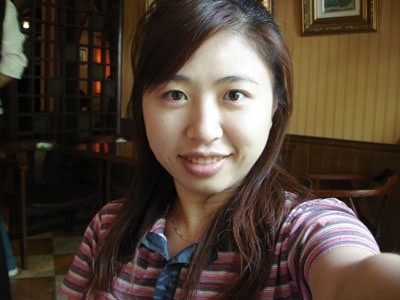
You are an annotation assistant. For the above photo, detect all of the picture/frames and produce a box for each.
[138,0,274,19]
[301,0,377,34]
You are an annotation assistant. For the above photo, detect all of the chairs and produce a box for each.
[304,167,397,252]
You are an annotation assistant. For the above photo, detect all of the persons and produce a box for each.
[56,0,400,300]
[1,0,30,279]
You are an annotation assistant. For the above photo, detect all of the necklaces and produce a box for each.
[169,211,199,244]
